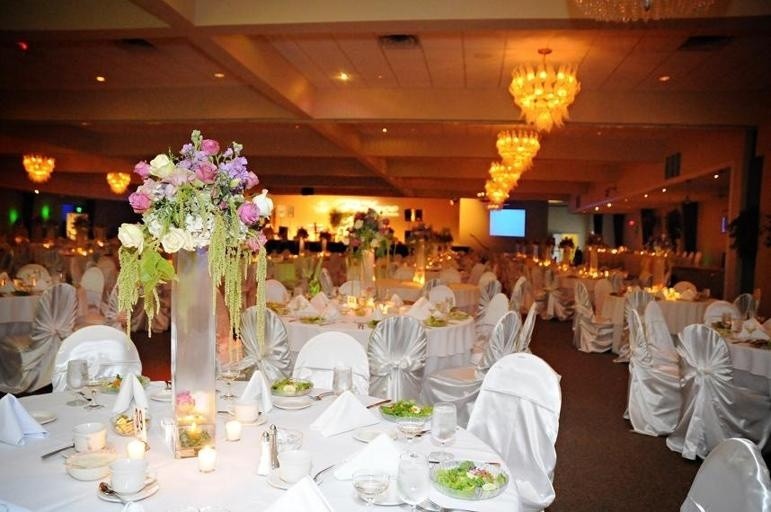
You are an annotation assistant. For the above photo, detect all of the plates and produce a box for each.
[27,410,57,425]
[60,354,513,512]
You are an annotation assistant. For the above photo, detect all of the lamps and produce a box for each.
[484,128,542,205]
[106,169,131,195]
[22,152,55,185]
[508,47,581,133]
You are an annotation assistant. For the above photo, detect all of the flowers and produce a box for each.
[346,208,399,277]
[113,130,274,362]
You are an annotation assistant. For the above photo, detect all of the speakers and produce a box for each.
[404,208,414,221]
[301,188,314,195]
[415,209,424,222]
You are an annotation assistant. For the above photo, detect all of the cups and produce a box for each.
[1,270,66,294]
[709,308,771,349]
[286,292,468,329]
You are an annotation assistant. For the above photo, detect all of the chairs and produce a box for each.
[664,437,770,512]
[240,278,538,429]
[320,243,502,315]
[2,236,174,393]
[493,251,770,462]
[4,326,562,511]
